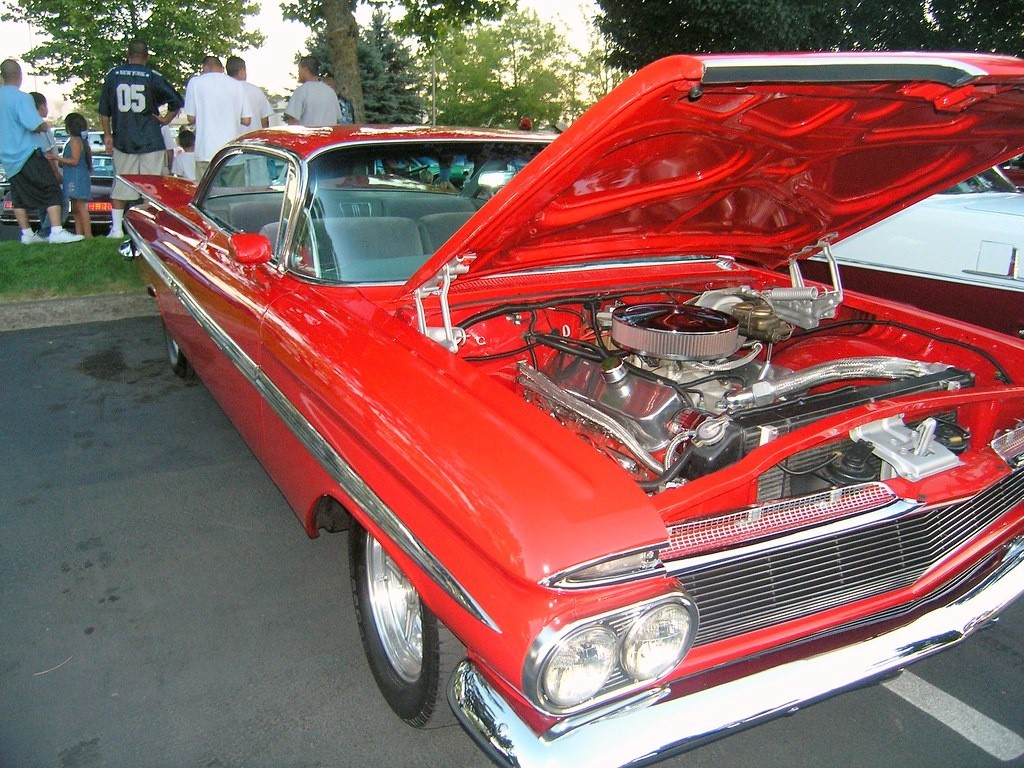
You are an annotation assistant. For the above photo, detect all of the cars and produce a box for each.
[0,28,1024,768]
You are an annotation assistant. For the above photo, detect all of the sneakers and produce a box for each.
[48,229,85,244]
[105,229,124,239]
[20,233,43,243]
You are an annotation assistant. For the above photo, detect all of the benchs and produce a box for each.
[221,195,487,235]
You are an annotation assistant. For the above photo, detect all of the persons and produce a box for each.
[182,56,251,187]
[283,55,343,126]
[43,112,94,241]
[159,124,175,176]
[0,59,85,243]
[171,129,197,183]
[28,91,72,241]
[224,55,275,134]
[98,39,185,238]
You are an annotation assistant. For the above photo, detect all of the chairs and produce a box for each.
[423,211,487,261]
[261,216,428,279]
